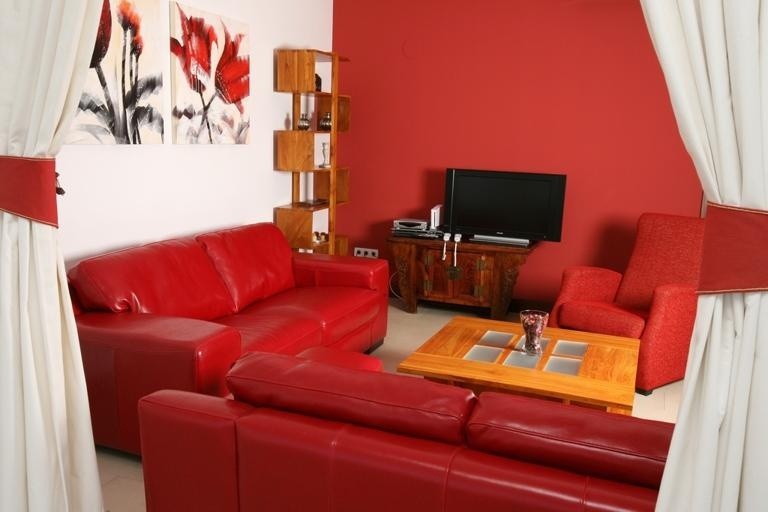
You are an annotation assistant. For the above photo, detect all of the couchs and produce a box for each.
[547,212,706,397]
[66,222,389,456]
[138,345,676,512]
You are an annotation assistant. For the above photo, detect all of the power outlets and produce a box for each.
[354,247,379,259]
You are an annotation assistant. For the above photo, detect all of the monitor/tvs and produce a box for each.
[444,168,567,248]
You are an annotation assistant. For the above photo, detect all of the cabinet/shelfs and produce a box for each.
[273,48,350,255]
[386,226,540,320]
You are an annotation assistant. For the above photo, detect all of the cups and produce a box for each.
[520,310,549,356]
